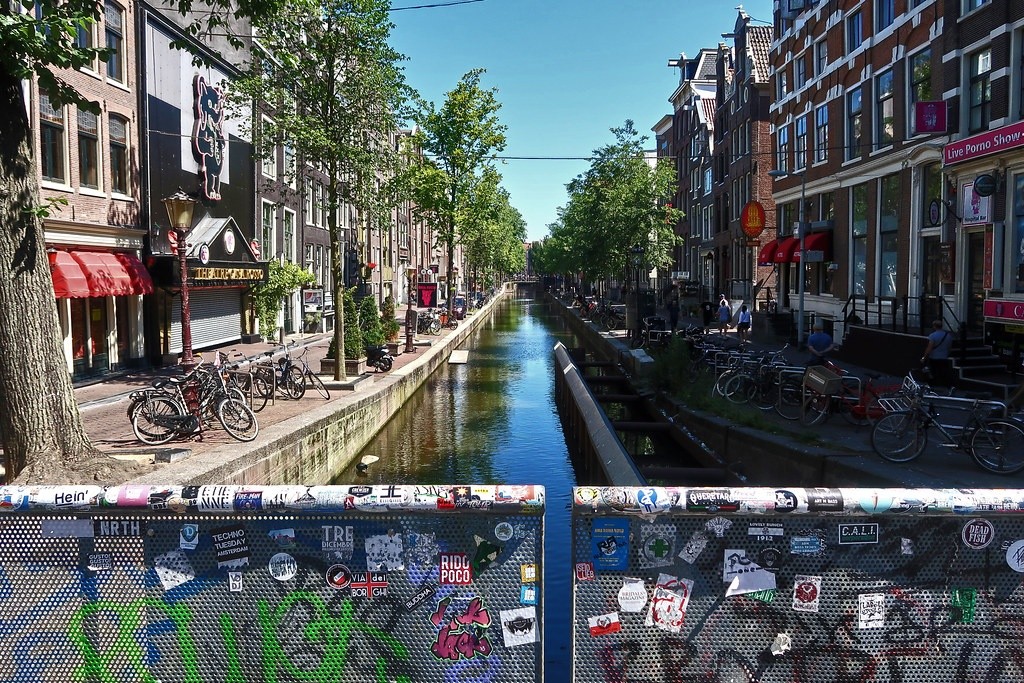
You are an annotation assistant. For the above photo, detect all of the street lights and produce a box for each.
[404,265,417,353]
[160,188,212,435]
[452,269,458,321]
[629,242,646,348]
[768,170,807,351]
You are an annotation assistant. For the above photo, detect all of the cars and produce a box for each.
[447,296,467,321]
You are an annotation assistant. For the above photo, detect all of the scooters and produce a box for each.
[365,343,395,374]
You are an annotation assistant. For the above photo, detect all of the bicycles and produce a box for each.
[418,307,459,336]
[127,338,331,446]
[570,291,1023,476]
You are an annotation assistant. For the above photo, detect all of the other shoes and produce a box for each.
[948,386,956,397]
[739,343,743,346]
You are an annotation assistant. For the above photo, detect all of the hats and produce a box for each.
[719,294,725,297]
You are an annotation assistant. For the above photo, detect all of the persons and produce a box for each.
[593,285,957,400]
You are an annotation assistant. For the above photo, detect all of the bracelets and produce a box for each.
[922,357,925,360]
[823,351,824,353]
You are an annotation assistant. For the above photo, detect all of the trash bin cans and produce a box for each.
[645,316,666,343]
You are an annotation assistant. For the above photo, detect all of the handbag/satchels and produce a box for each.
[920,351,929,362]
[727,308,732,323]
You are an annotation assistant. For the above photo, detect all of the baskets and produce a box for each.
[803,365,842,395]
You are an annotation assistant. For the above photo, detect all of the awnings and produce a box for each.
[757,233,829,265]
[50,249,154,300]
